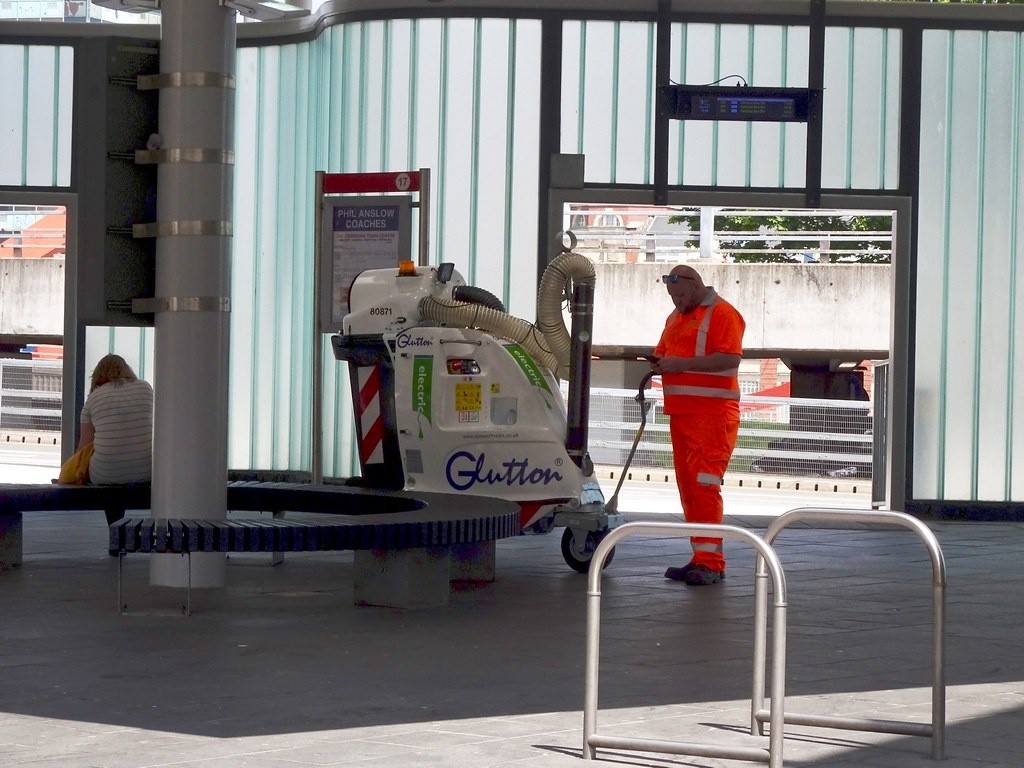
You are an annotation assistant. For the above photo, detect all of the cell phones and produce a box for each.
[644,354,660,365]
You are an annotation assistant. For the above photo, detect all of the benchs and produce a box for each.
[1,481,523,613]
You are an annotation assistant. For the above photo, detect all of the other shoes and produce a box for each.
[664,561,726,580]
[685,562,721,585]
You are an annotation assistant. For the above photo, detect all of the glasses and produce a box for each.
[662,275,696,283]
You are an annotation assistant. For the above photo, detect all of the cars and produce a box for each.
[748,434,872,479]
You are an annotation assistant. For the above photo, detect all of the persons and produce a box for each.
[76,354,153,554]
[649,265,746,584]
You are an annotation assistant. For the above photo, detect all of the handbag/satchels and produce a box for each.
[58,441,94,484]
